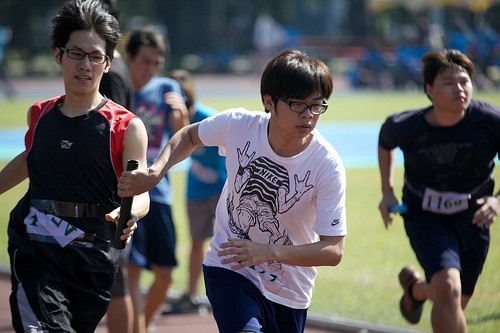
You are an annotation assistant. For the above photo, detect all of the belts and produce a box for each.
[28,200,106,218]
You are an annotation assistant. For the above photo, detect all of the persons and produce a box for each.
[1,0,150,333]
[378,50,500,333]
[126,28,190,333]
[161,84,227,315]
[117,51,347,333]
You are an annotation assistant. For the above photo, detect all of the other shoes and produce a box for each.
[399,265,426,324]
[161,295,208,315]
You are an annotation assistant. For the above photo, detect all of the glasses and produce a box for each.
[280,97,328,115]
[60,47,109,65]
